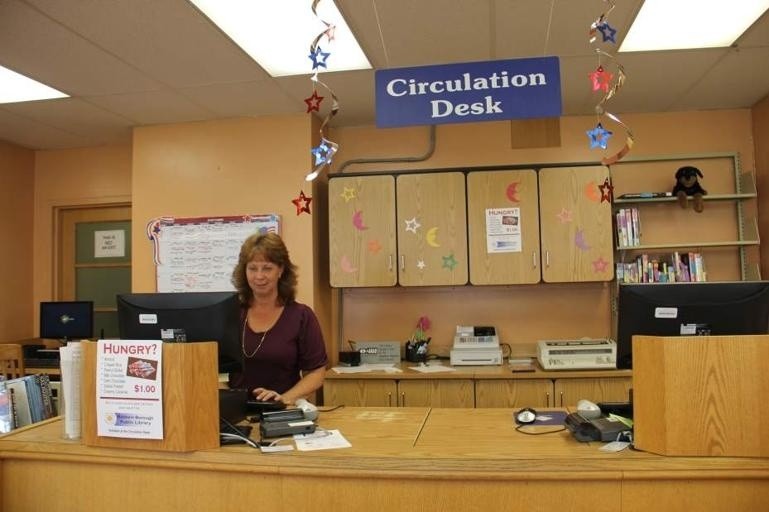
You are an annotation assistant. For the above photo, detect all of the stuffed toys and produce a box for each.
[671,166,706,213]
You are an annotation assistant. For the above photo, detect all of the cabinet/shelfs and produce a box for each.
[476,378,629,410]
[467,165,612,287]
[325,168,467,289]
[320,377,474,409]
[614,151,769,288]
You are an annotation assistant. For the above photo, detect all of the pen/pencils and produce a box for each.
[408,335,431,349]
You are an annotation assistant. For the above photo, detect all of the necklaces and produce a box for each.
[241,304,280,358]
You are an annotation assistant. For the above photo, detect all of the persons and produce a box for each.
[226,230,331,407]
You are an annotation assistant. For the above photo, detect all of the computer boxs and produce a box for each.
[218,387,249,432]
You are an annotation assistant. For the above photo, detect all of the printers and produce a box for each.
[537,338,617,372]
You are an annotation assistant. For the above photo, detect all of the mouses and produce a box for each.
[517,408,536,423]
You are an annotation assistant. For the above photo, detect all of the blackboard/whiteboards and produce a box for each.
[155,214,279,293]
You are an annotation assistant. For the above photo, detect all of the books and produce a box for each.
[615,208,706,282]
[0,371,54,434]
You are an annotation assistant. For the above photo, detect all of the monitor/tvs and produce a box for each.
[116,291,244,373]
[616,280,769,371]
[39,301,93,339]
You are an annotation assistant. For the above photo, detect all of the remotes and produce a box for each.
[508,358,533,363]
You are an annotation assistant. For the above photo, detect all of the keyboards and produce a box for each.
[247,398,286,414]
